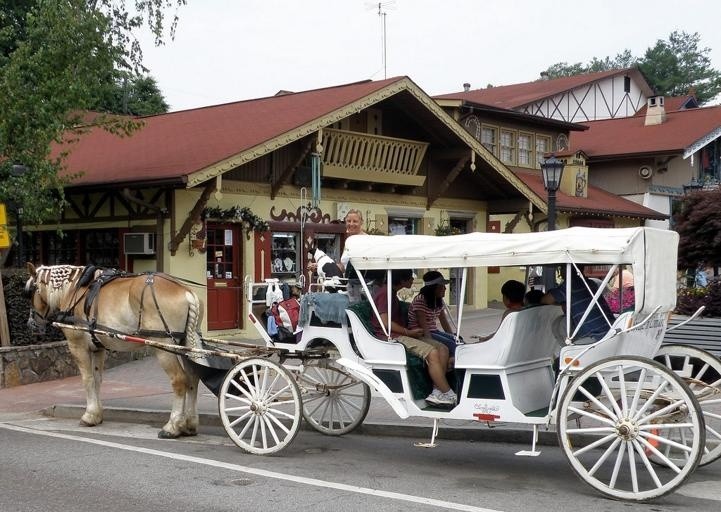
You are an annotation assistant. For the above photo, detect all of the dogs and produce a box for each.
[305,238,348,295]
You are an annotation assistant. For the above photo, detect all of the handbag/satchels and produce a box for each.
[267,282,302,336]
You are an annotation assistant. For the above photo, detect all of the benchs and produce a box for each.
[346,299,456,406]
[548,308,674,381]
[453,303,557,416]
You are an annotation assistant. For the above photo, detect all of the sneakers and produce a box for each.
[425,393,457,409]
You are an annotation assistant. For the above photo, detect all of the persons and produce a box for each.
[371,270,458,408]
[308,207,371,292]
[605,263,632,290]
[683,265,709,288]
[477,265,619,344]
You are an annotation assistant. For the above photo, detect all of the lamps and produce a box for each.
[273,231,297,238]
[315,233,337,240]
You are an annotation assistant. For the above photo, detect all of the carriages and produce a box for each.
[21,226,721,503]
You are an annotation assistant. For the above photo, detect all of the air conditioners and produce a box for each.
[123,230,155,255]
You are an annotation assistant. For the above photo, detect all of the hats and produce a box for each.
[424,276,450,285]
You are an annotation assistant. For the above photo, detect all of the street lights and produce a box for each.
[537,152,568,289]
[680,175,706,286]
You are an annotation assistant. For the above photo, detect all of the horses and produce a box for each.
[26,261,205,440]
